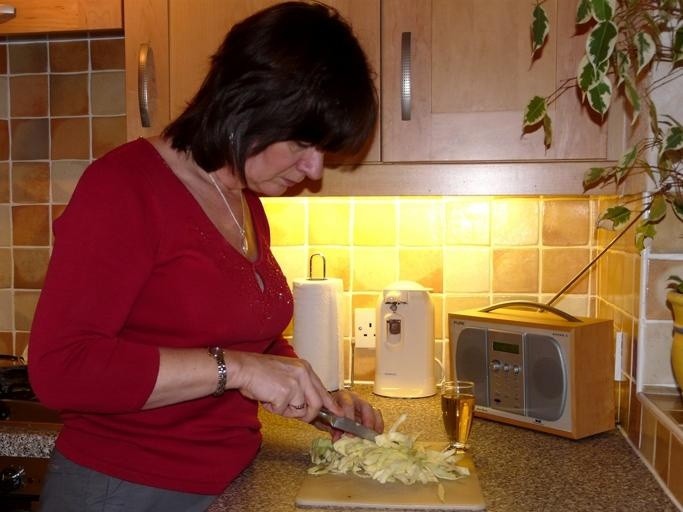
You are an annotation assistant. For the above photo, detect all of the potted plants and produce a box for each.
[521,0,683,401]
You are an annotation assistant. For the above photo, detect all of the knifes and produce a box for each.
[318,407,381,443]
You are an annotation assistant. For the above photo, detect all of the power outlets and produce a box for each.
[354,307,376,348]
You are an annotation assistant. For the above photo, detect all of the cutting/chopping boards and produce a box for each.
[296,433,486,512]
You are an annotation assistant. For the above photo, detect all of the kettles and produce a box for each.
[371,280,439,399]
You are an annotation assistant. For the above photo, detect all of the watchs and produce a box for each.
[207,342,229,398]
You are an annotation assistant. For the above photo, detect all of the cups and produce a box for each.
[442,380,476,455]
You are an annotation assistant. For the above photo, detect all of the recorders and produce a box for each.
[448,204,656,441]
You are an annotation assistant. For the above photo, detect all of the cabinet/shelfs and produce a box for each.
[380,1,608,162]
[124,1,382,163]
[1,1,124,37]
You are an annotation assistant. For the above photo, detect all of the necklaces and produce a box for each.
[207,173,250,258]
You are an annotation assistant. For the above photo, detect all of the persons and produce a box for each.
[26,2,379,512]
